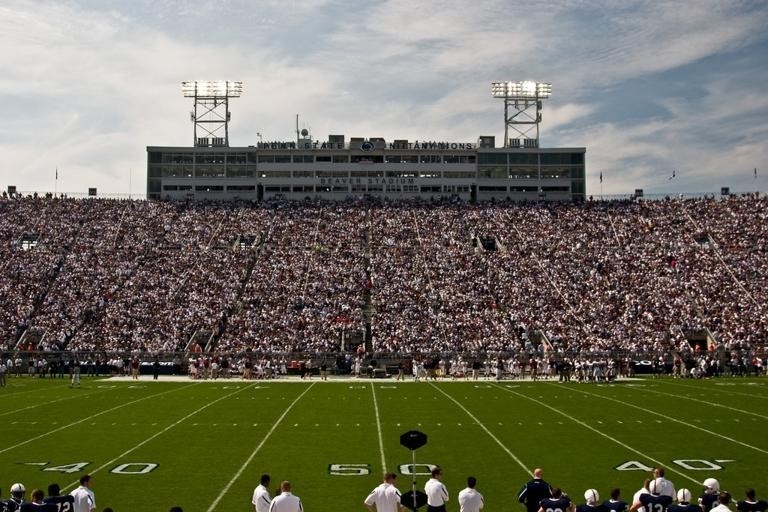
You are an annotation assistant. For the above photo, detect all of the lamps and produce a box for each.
[490,80,553,149]
[180,79,243,147]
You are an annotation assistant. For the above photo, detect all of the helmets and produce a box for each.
[702,477,720,494]
[11,482,25,500]
[585,488,599,504]
[677,488,691,503]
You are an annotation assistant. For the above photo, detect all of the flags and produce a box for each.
[667,171,678,182]
[753,166,758,181]
[599,170,603,183]
[55,169,58,180]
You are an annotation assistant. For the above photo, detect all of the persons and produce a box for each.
[458,477,484,512]
[424,468,449,512]
[631,468,767,512]
[516,468,629,512]
[365,473,406,512]
[252,475,302,512]
[0,186,768,388]
[2,475,96,512]
